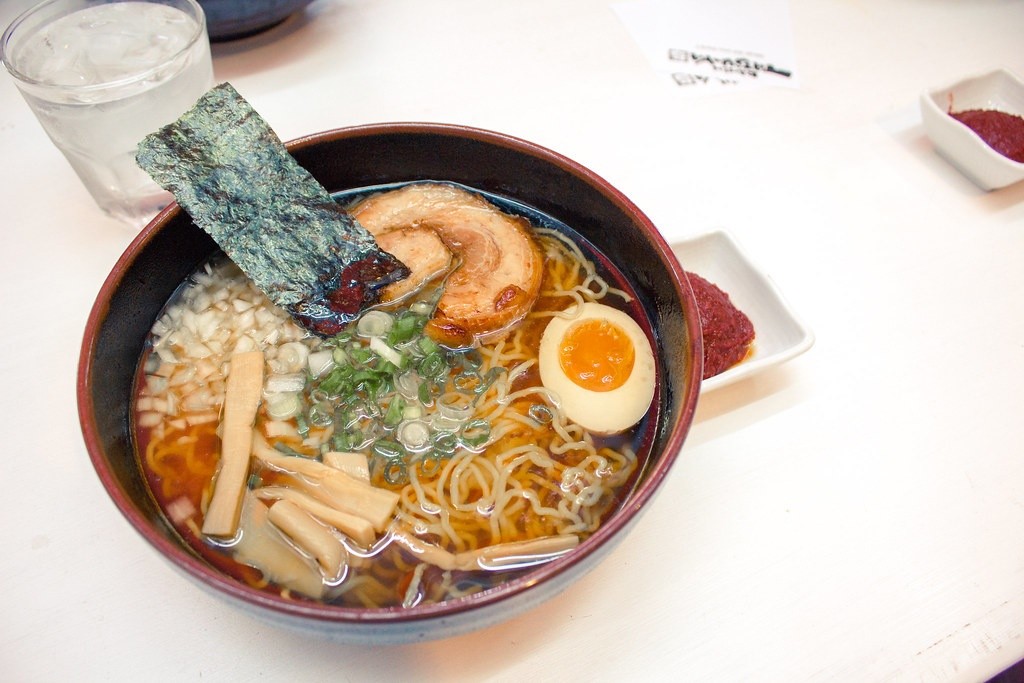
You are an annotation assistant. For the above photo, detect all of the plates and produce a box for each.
[916,65,1024,191]
[670,231,817,395]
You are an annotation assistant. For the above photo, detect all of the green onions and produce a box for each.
[260,297,554,486]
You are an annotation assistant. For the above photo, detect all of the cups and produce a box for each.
[0,1,216,227]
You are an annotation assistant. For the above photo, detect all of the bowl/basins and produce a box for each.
[76,122,704,645]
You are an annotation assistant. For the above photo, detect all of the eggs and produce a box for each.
[537,302,655,433]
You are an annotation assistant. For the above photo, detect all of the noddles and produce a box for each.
[129,182,661,613]
